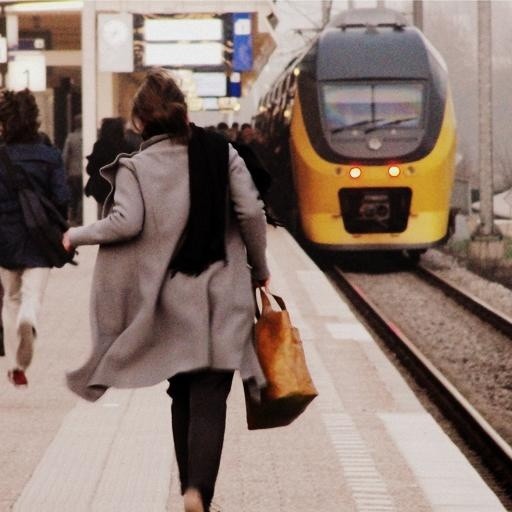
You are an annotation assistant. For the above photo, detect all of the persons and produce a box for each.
[118,40,169,117]
[0,89,71,387]
[62,73,270,512]
[62,114,82,225]
[207,112,288,217]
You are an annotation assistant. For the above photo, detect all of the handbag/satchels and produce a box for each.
[243,286,319,431]
[18,186,78,268]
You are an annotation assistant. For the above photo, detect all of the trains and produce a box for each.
[252,6,458,271]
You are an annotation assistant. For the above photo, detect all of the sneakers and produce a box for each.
[8,322,37,388]
